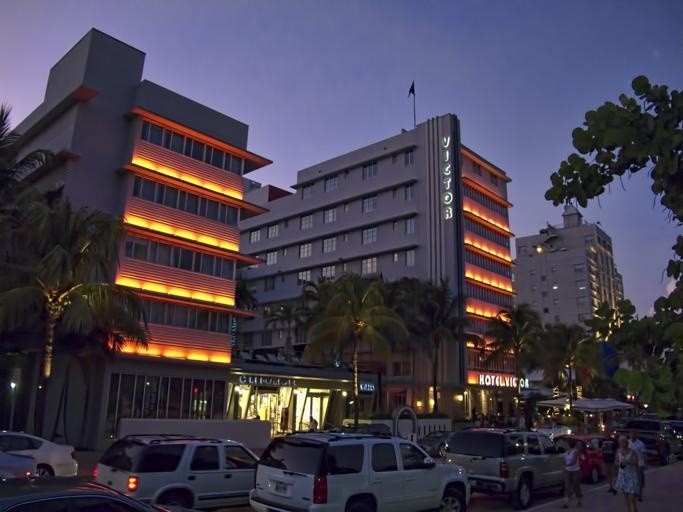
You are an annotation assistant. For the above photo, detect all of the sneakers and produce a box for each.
[561,504,568,509]
[608,487,617,495]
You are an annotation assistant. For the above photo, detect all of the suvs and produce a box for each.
[440,428,566,509]
[250,429,472,511]
[94,434,261,512]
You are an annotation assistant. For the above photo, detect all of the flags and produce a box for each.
[407,80,415,96]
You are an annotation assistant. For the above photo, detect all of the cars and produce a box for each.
[0,451,36,480]
[0,430,78,478]
[669,421,683,432]
[552,434,620,484]
[0,476,204,512]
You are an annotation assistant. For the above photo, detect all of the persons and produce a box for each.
[598,431,618,496]
[467,405,651,431]
[560,440,583,508]
[310,416,319,431]
[613,436,642,511]
[627,430,647,502]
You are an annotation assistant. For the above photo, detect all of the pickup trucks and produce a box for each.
[614,419,683,465]
